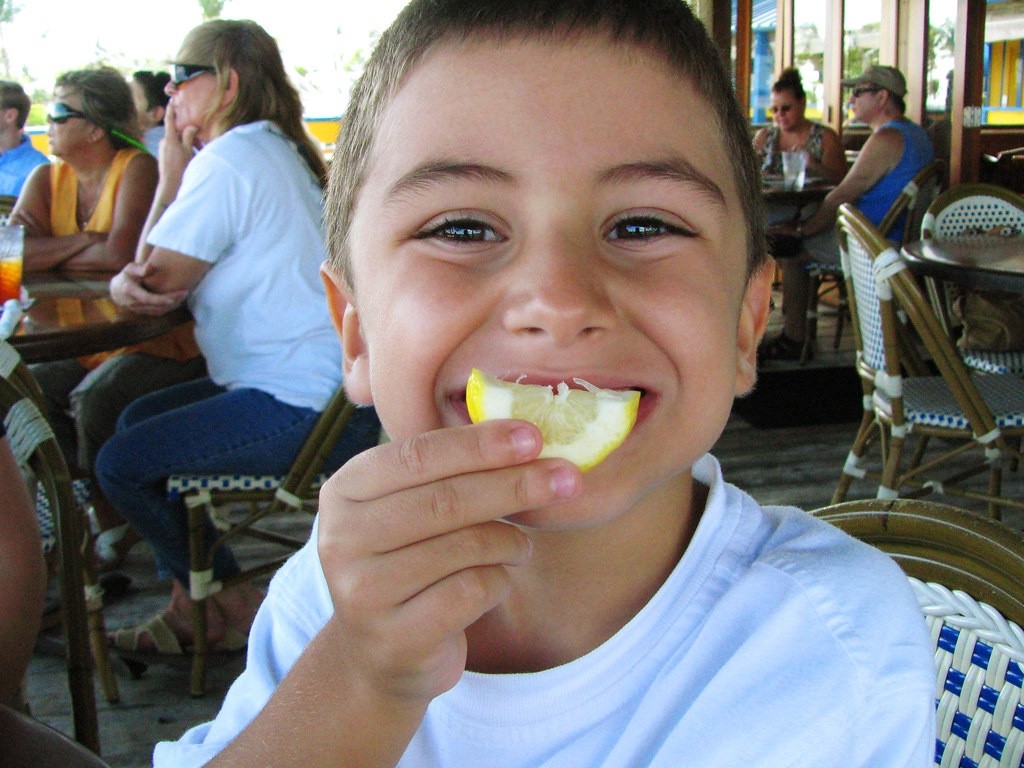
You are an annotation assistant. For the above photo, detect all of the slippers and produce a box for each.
[107,610,250,669]
[93,523,142,574]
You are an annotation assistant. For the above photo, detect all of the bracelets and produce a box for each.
[797,223,807,239]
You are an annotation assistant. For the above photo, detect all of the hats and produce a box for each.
[843,65,909,96]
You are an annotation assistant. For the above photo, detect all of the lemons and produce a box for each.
[466,368,641,474]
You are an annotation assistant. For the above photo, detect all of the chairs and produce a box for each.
[797,158,947,368]
[806,499,1024,768]
[167,379,359,699]
[800,183,1024,520]
[0,339,121,760]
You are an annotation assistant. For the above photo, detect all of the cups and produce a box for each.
[0,225,23,305]
[782,151,806,192]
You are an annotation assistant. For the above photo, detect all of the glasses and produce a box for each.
[852,87,884,96]
[46,100,89,123]
[768,103,796,113]
[166,59,217,83]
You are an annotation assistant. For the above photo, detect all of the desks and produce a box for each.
[900,234,1024,293]
[0,269,193,755]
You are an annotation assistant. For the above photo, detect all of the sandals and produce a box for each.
[758,330,815,364]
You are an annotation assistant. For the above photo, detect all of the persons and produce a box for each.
[751,65,934,360]
[0,68,203,568]
[152,1,935,768]
[93,18,382,662]
[0,434,48,716]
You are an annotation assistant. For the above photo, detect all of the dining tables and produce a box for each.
[758,173,839,201]
